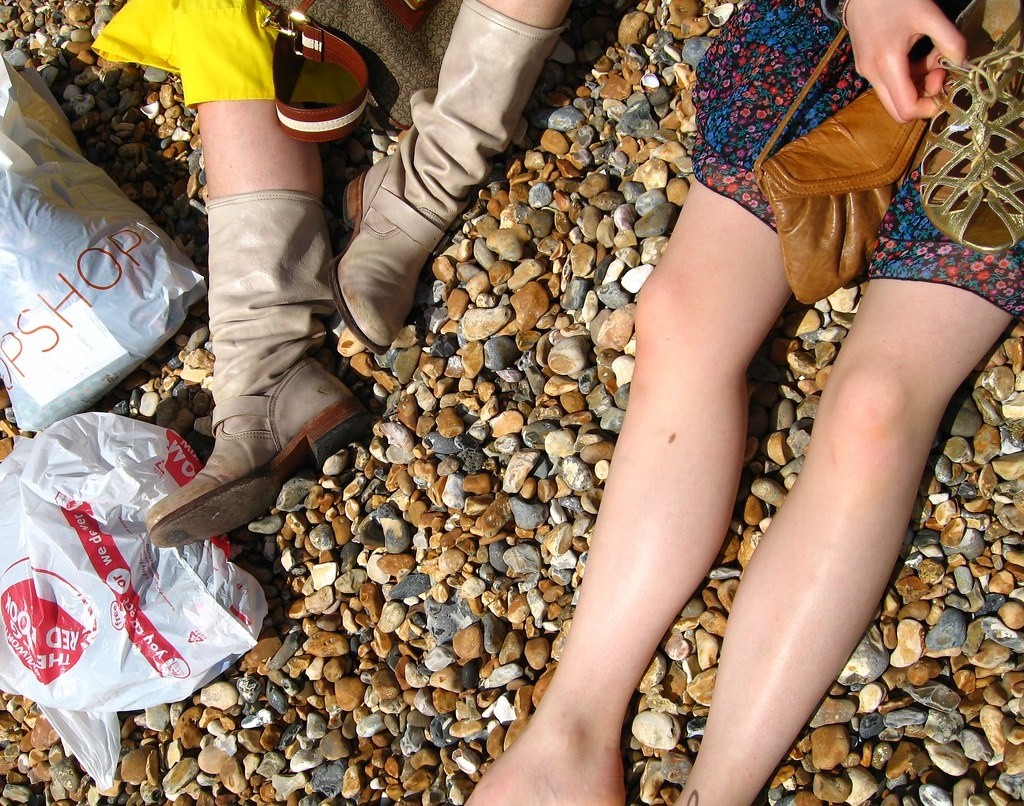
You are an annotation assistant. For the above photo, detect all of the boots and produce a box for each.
[145,190,369,550]
[333,0,569,352]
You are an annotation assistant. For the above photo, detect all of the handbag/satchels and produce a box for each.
[252,0,464,141]
[763,88,930,306]
[0,51,208,431]
[0,414,268,791]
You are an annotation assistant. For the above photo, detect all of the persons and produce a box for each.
[456,0,1024,806]
[89,0,574,547]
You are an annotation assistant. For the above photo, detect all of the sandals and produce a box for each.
[919,0,1024,255]
